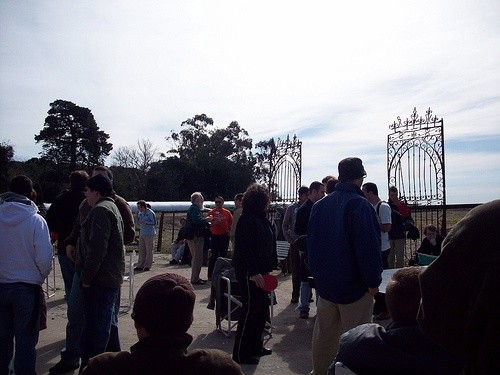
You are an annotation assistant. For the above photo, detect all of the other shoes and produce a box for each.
[202,279,206,282]
[49,358,79,374]
[144,267,149,271]
[191,280,206,285]
[134,266,143,270]
[309,297,314,303]
[291,296,299,303]
[299,311,309,319]
[243,356,260,364]
[170,259,178,265]
[258,346,272,356]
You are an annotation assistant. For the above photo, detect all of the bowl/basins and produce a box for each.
[258,275,278,291]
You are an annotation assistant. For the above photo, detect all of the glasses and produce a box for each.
[215,201,222,203]
[131,312,135,320]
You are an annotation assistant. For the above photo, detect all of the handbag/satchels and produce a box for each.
[401,213,414,231]
[180,228,194,240]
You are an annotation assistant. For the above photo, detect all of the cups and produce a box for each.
[215,211,220,219]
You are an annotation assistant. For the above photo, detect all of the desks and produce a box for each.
[309,266,429,304]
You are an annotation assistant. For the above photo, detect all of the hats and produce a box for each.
[134,273,196,336]
[339,158,366,181]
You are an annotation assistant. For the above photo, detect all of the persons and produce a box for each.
[232,185,278,365]
[363,183,392,320]
[170,217,186,264]
[76,174,125,375]
[45,171,90,296]
[82,273,244,375]
[307,158,383,375]
[416,202,500,375]
[282,186,314,304]
[409,224,443,267]
[327,268,436,375]
[231,193,243,243]
[0,175,53,375]
[186,192,212,284]
[208,196,233,281]
[388,187,411,267]
[295,182,324,319]
[134,200,157,272]
[322,176,338,194]
[50,166,135,374]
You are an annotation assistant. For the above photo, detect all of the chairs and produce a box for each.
[41,243,56,299]
[276,241,290,276]
[118,250,137,314]
[217,257,274,339]
